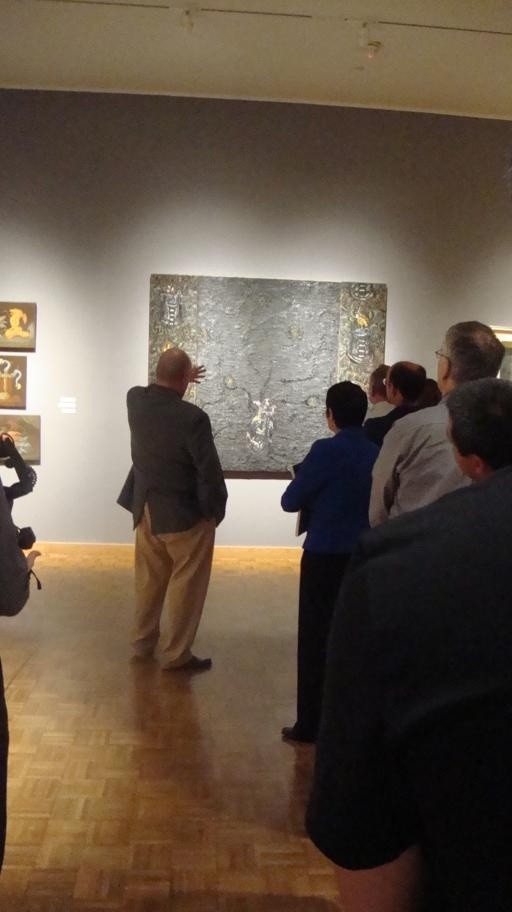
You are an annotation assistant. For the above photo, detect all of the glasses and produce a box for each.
[382,378,392,389]
[435,349,451,363]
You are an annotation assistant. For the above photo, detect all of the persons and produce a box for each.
[366,362,429,446]
[369,322,508,530]
[444,375,512,484]
[0,433,40,878]
[115,348,228,673]
[301,467,512,912]
[281,379,382,743]
[362,365,397,425]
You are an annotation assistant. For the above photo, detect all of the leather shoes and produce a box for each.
[164,656,209,672]
[283,723,312,739]
